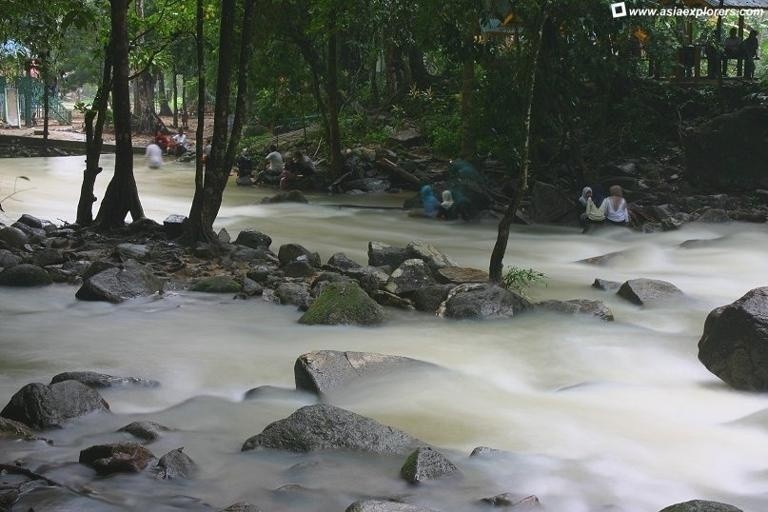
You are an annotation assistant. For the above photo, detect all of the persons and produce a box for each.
[407,183,438,220]
[736,30,759,81]
[723,25,742,76]
[145,123,317,190]
[436,187,460,222]
[575,185,605,225]
[630,24,644,58]
[599,186,629,226]
